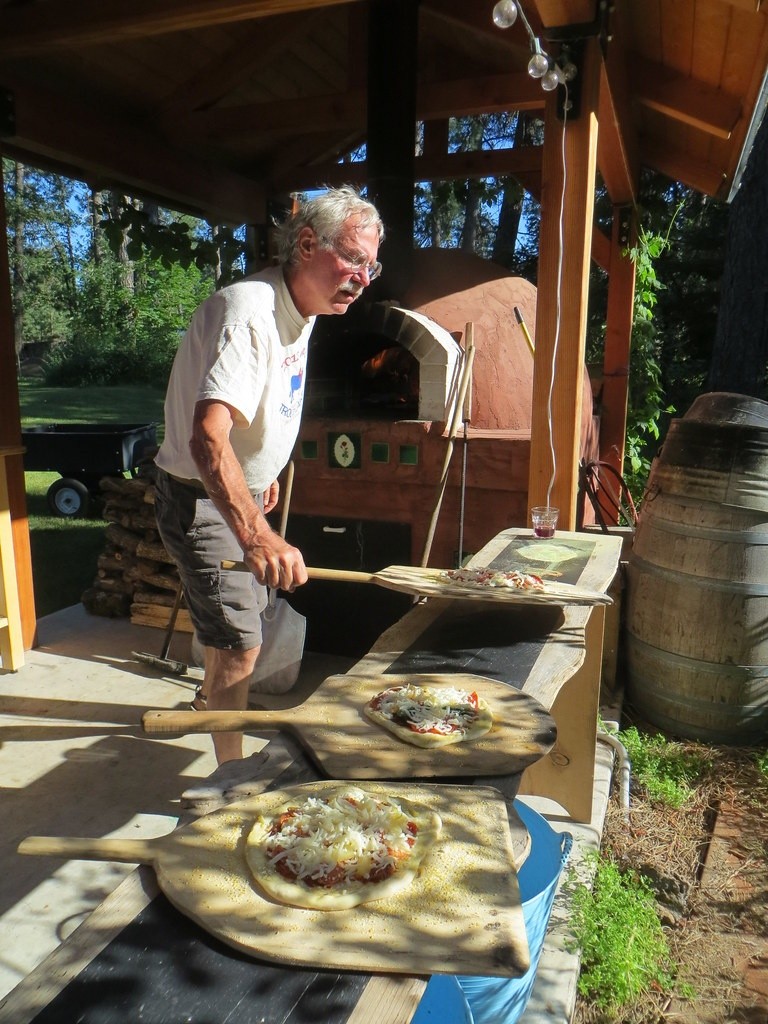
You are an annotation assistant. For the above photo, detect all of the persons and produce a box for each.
[144,185,385,770]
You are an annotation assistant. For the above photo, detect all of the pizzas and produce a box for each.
[363,685,493,749]
[437,568,547,595]
[243,785,442,912]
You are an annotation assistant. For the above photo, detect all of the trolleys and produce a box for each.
[21,423,157,518]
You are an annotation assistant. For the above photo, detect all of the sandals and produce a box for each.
[190,684,267,712]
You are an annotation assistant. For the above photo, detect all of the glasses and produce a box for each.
[313,230,382,282]
